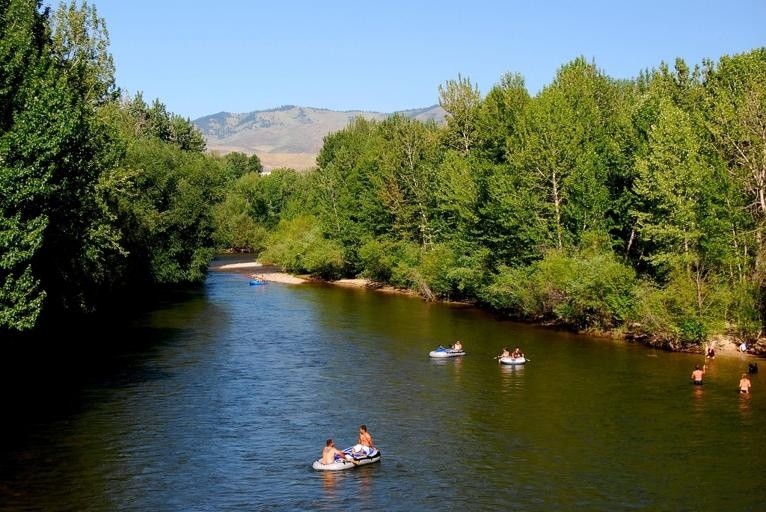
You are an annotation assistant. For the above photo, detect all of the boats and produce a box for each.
[498,356,531,365]
[429,344,466,358]
[312,444,381,470]
[249,280,266,285]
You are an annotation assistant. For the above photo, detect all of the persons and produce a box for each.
[321,438,345,465]
[691,364,706,385]
[739,372,752,394]
[512,346,525,358]
[451,340,463,350]
[706,347,715,359]
[498,347,510,358]
[351,424,380,454]
